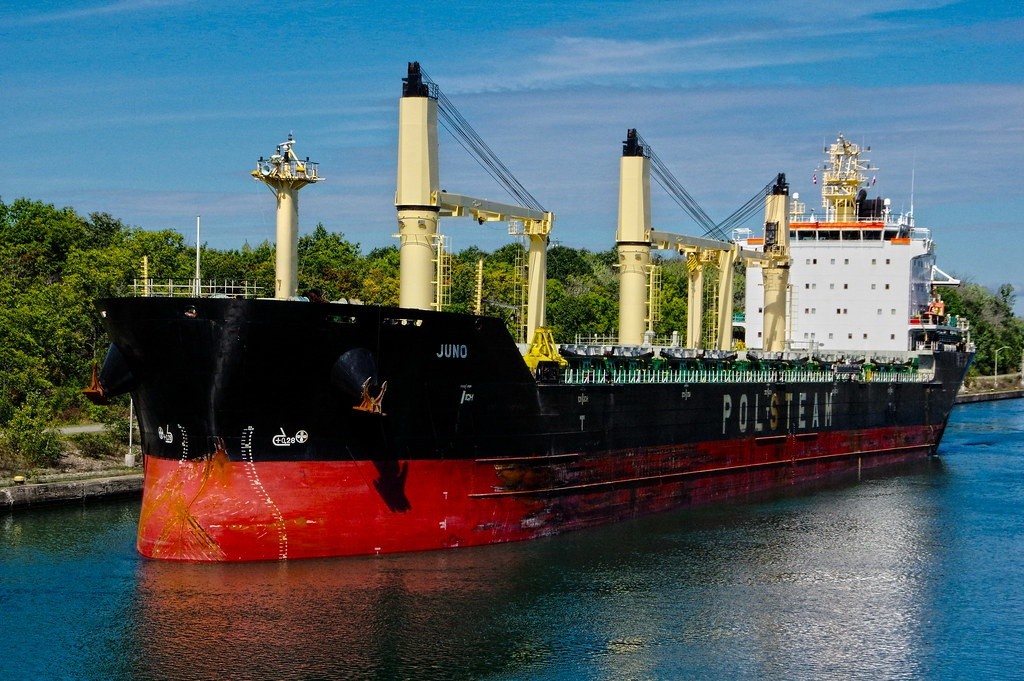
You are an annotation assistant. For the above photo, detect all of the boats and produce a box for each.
[81,57,979,566]
